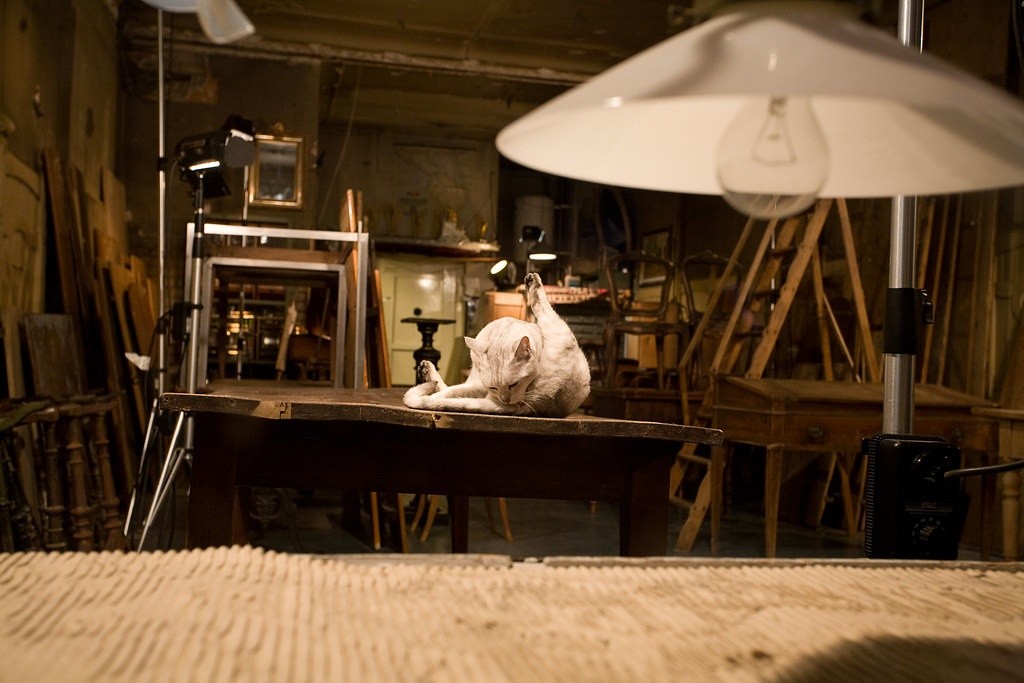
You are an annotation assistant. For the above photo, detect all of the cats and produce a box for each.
[402,271,592,417]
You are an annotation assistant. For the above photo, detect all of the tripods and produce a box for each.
[123,4,204,554]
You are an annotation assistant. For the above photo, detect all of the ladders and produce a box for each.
[668,200,882,554]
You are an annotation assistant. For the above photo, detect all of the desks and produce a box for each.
[124,384,729,553]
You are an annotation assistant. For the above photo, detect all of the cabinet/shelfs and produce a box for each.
[710,379,1003,557]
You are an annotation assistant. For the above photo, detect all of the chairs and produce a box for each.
[602,248,697,388]
[682,246,772,387]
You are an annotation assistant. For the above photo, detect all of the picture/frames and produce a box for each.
[242,130,309,212]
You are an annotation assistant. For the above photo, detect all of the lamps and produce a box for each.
[523,224,558,261]
[495,0,1024,217]
[489,259,512,291]
